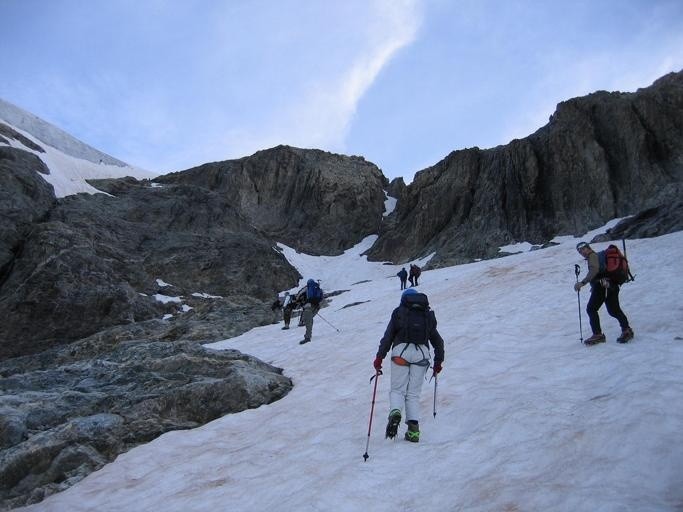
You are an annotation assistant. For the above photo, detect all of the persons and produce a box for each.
[574,242,634,345]
[397,264,421,290]
[283,291,292,330]
[374,289,445,441]
[296,279,319,344]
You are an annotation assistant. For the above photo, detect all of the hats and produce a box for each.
[401,289,417,304]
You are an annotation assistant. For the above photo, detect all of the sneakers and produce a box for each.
[300,338,311,344]
[584,333,606,345]
[281,327,290,330]
[617,328,634,344]
[385,409,402,440]
[405,430,420,442]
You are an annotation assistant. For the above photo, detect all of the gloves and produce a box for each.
[433,360,443,377]
[374,356,382,371]
[574,282,584,291]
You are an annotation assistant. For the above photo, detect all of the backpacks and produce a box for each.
[400,293,430,345]
[307,282,321,301]
[586,244,629,286]
